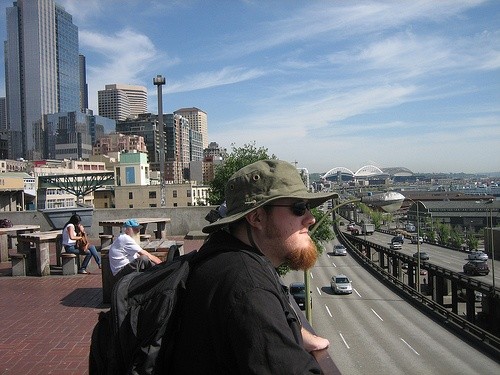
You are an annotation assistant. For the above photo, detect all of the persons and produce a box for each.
[62,214,101,273]
[157,158,340,375]
[109,220,161,281]
[300,327,329,352]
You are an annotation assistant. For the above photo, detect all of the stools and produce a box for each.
[10,229,167,276]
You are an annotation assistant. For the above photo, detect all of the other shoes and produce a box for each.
[78,268,87,274]
[99,264,102,269]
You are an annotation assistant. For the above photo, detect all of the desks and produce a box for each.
[101,240,185,304]
[17,230,82,276]
[99,217,171,239]
[0,224,40,263]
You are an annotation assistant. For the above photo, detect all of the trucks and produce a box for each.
[361,224,375,235]
[411,236,423,244]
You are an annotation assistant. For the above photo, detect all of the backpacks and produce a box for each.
[0,218,13,228]
[88,243,284,375]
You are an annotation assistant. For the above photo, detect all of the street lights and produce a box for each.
[152,74,167,185]
[303,190,406,327]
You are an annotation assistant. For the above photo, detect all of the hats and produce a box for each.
[201,158,340,236]
[123,218,144,229]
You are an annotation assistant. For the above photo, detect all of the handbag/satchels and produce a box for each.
[76,231,89,254]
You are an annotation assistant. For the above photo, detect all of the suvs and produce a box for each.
[289,282,312,310]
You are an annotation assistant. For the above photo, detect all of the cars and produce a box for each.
[390,242,402,250]
[346,221,355,231]
[467,251,489,261]
[420,270,427,275]
[339,222,345,226]
[463,260,490,275]
[351,228,359,236]
[401,262,416,275]
[391,236,404,244]
[330,274,352,294]
[413,251,430,260]
[333,244,347,256]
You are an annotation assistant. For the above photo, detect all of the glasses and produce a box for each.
[269,201,311,217]
